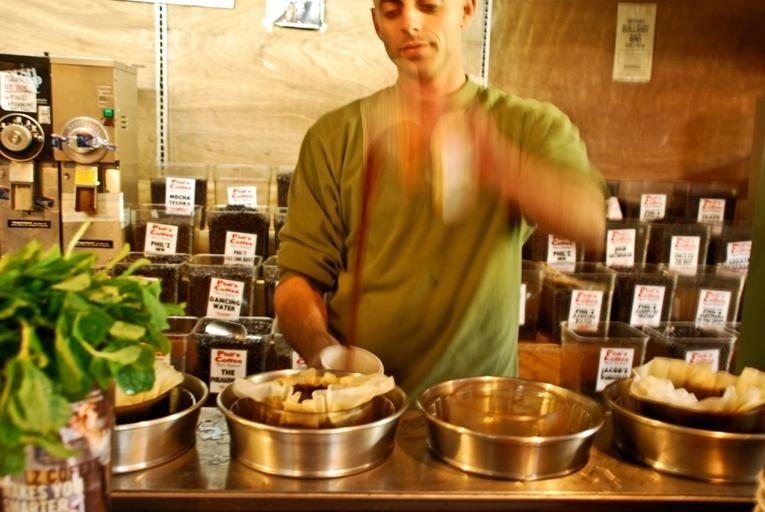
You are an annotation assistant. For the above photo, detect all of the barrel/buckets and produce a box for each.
[0,376,114,511]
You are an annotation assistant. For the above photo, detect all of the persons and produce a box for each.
[270,1,608,412]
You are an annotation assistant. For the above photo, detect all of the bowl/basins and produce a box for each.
[112,371,208,472]
[602,376,765,486]
[218,368,410,481]
[417,375,604,482]
